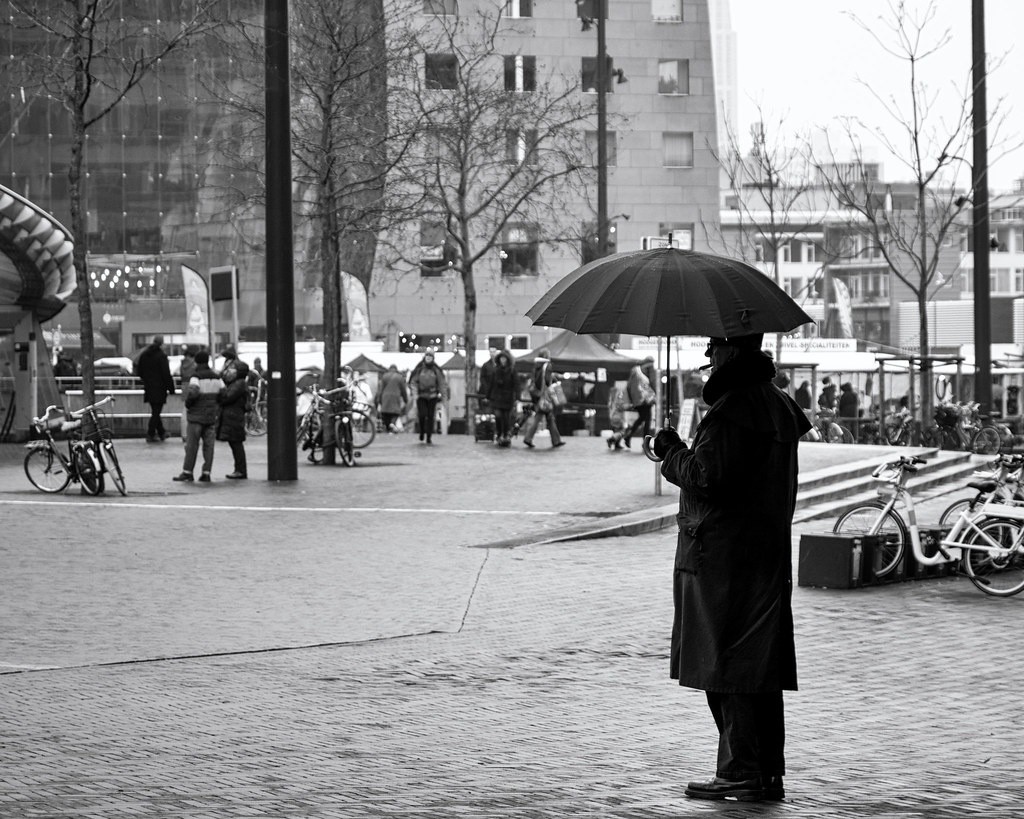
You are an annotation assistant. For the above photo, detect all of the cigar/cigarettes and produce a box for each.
[699,363,714,371]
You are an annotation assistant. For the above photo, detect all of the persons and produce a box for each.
[135,335,175,446]
[406,348,448,444]
[51,351,78,377]
[606,385,633,450]
[562,357,704,407]
[480,349,523,447]
[175,348,376,442]
[990,375,1024,418]
[794,376,875,445]
[653,327,812,806]
[374,365,409,434]
[625,365,661,451]
[477,346,502,406]
[172,352,227,483]
[519,346,560,450]
[213,360,254,478]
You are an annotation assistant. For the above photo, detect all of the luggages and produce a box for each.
[473,399,496,443]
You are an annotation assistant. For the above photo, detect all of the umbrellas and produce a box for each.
[524,328,646,403]
[524,247,818,463]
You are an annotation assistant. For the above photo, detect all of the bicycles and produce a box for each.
[296,365,376,467]
[243,368,268,437]
[24,405,102,497]
[69,396,128,497]
[832,455,1024,597]
[799,399,1024,563]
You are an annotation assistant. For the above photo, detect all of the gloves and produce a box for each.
[654,428,681,460]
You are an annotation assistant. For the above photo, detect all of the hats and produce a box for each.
[711,333,764,350]
[425,350,435,356]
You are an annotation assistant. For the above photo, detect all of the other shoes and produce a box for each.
[419,434,432,443]
[624,437,630,447]
[553,442,566,447]
[389,423,398,435]
[524,440,535,447]
[147,434,170,442]
[496,437,508,442]
[606,439,624,449]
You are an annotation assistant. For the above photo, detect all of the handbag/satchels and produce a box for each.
[539,386,553,412]
[547,376,568,405]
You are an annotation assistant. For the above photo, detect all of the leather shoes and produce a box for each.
[226,472,246,479]
[173,472,193,481]
[199,474,211,482]
[770,776,785,801]
[685,776,771,802]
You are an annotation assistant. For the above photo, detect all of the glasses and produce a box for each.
[707,343,722,350]
